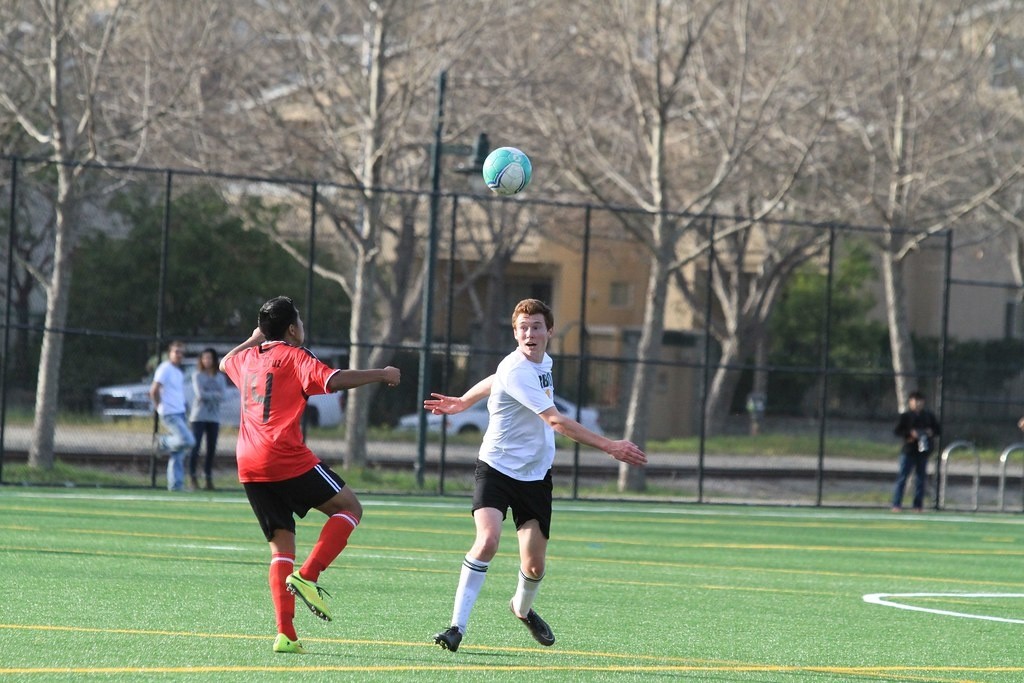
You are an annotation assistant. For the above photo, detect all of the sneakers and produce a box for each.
[433,626,462,652]
[510,598,555,646]
[274,633,307,654]
[286,570,334,622]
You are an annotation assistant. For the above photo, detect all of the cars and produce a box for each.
[92,360,346,424]
[395,392,607,436]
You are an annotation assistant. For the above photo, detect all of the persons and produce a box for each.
[188,348,226,491]
[150,339,197,492]
[890,392,942,513]
[219,296,400,654]
[423,298,647,651]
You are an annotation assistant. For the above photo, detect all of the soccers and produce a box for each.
[482,146,533,196]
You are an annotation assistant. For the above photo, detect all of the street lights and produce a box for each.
[413,70,493,488]
